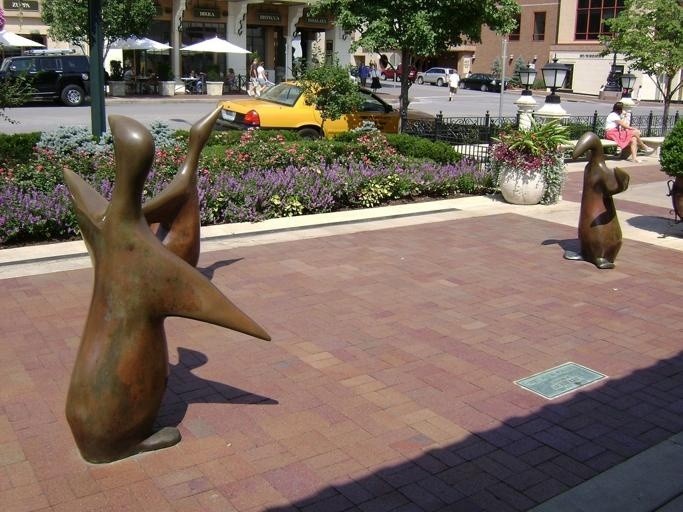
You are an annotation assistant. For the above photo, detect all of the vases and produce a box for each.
[498,160,547,205]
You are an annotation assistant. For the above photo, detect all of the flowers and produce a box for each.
[478,112,571,206]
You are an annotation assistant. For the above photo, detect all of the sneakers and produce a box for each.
[247,90,260,97]
[631,148,654,164]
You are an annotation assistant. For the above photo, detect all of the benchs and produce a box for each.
[638,135,667,158]
[560,136,619,159]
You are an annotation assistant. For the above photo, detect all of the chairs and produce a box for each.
[123,74,241,97]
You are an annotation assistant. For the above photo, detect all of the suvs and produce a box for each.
[416,66,459,86]
[0,49,91,106]
[380,64,417,82]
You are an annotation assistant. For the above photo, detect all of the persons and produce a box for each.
[123,56,271,96]
[359,59,382,94]
[447,69,459,103]
[603,102,655,164]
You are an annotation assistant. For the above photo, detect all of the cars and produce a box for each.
[457,73,508,92]
[212,79,448,144]
[350,66,371,77]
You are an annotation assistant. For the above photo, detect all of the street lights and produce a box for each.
[512,59,537,132]
[533,53,569,204]
[615,66,635,155]
[596,28,625,101]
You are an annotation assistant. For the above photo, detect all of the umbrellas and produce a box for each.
[105,35,253,65]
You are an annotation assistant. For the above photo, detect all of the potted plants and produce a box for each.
[655,114,682,226]
